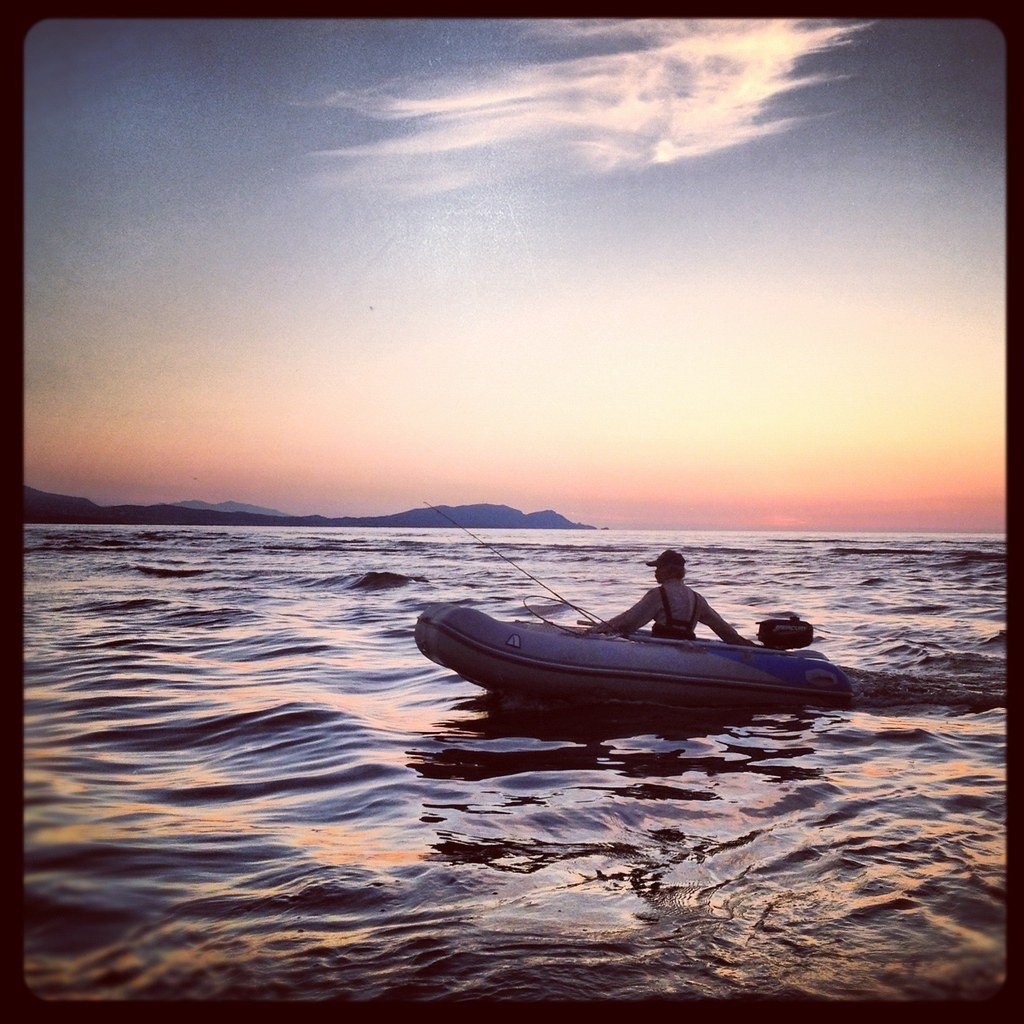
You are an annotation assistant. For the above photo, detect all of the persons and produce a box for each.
[584,549,755,646]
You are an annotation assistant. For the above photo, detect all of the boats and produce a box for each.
[413,602,853,709]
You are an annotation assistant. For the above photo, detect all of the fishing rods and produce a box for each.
[425,501,600,629]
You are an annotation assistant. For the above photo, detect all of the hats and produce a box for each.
[646,550,687,567]
